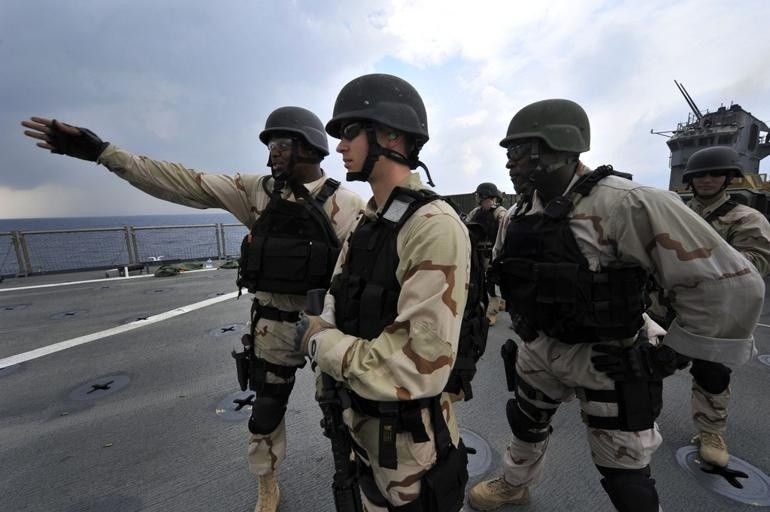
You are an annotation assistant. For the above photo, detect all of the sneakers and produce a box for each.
[699,431,729,468]
[469,477,532,510]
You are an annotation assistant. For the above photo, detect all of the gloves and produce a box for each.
[45,119,109,162]
[295,295,335,356]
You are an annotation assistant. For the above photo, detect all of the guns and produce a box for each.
[309,289,363,512]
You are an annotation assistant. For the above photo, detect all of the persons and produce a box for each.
[642,147,770,469]
[468,184,507,326]
[297,72,475,512]
[468,99,766,512]
[16,103,370,511]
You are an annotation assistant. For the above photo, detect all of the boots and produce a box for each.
[254,477,280,512]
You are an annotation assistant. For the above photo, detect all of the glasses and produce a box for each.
[338,120,368,140]
[690,170,730,177]
[507,140,542,160]
[268,137,296,152]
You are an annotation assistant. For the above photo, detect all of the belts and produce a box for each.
[349,390,441,414]
[540,322,639,344]
[258,307,300,322]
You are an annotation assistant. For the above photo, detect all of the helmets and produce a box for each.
[259,107,329,155]
[500,99,590,153]
[473,183,498,197]
[682,146,745,184]
[326,74,429,147]
